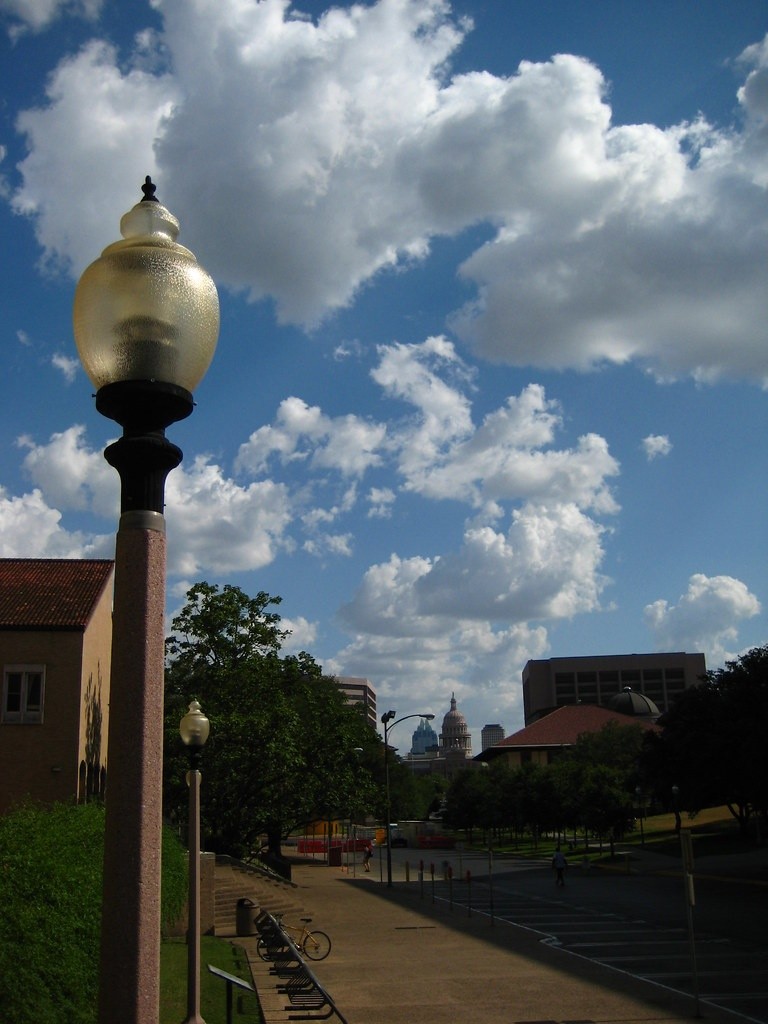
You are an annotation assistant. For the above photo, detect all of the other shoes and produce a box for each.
[560,883,565,886]
[556,881,559,884]
[365,870,370,872]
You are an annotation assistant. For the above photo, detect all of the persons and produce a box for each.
[552,847,569,886]
[353,826,356,838]
[363,842,373,871]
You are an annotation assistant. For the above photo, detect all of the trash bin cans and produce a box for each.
[236,897,261,938]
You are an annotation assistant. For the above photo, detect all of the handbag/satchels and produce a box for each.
[563,865,567,873]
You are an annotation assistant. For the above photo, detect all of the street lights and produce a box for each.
[635,785,645,844]
[180,696,209,1024]
[69,174,221,1024]
[381,710,435,888]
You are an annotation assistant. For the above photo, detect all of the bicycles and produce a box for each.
[256,914,332,961]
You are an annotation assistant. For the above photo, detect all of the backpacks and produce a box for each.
[365,847,374,858]
